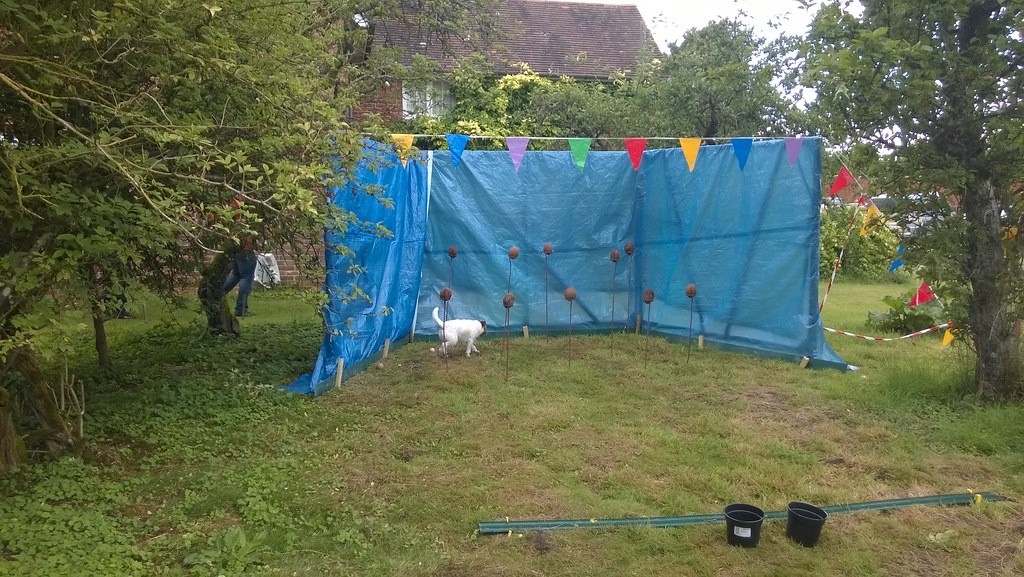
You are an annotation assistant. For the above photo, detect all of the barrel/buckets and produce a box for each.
[723,503,765,549]
[787,501,827,548]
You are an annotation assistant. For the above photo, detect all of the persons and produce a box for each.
[102,261,136,318]
[222,189,257,315]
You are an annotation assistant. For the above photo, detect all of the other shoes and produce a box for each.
[235,310,250,316]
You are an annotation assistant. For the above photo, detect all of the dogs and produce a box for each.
[432,307,487,358]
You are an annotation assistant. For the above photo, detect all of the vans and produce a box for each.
[819,191,966,237]
